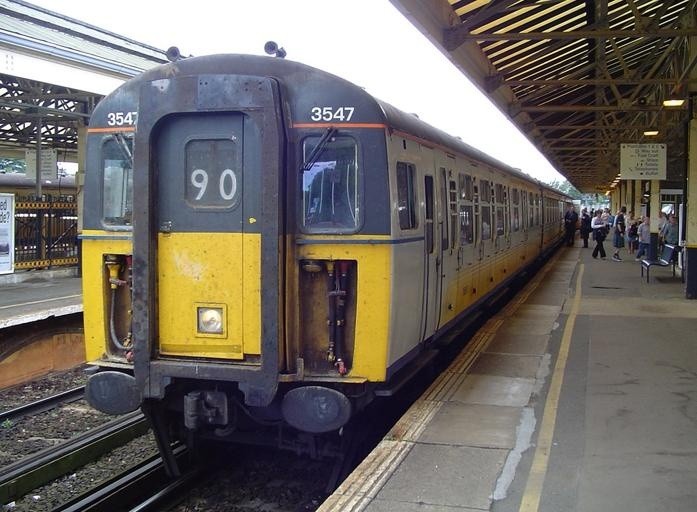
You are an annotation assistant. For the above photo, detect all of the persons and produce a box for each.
[590,210,607,260]
[583,207,619,228]
[624,211,637,254]
[658,211,678,263]
[611,207,626,262]
[564,205,578,248]
[579,207,589,248]
[634,216,650,262]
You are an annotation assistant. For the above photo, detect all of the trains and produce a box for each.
[78,40,574,496]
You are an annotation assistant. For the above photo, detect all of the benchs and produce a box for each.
[641,244,682,283]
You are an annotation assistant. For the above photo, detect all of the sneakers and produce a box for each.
[612,256,621,262]
[582,246,589,248]
[635,257,642,262]
[593,256,600,260]
[617,258,625,262]
[601,256,610,260]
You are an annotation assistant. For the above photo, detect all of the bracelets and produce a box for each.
[618,230,623,234]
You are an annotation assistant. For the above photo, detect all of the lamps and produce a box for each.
[643,127,659,136]
[663,92,686,106]
